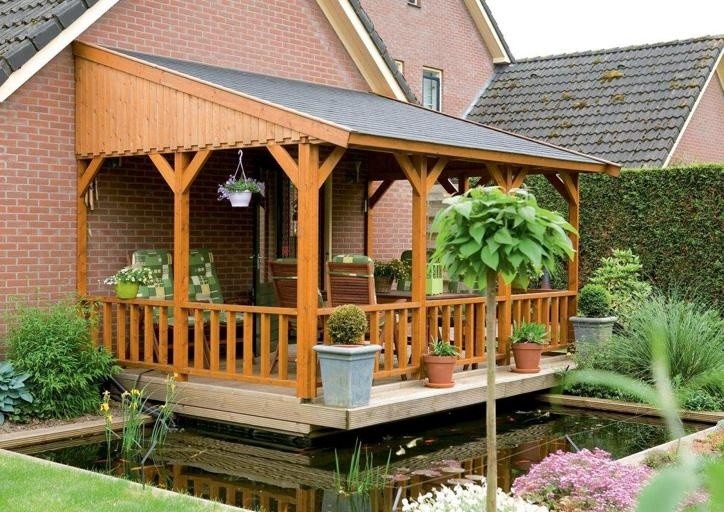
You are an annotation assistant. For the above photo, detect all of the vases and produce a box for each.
[228,190,252,207]
[115,280,138,299]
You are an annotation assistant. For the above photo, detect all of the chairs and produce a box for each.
[130,247,408,382]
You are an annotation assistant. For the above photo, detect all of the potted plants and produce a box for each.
[567,283,619,351]
[420,334,461,389]
[507,315,550,373]
[312,302,383,408]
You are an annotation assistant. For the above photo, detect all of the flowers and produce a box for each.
[101,266,158,288]
[216,174,265,201]
